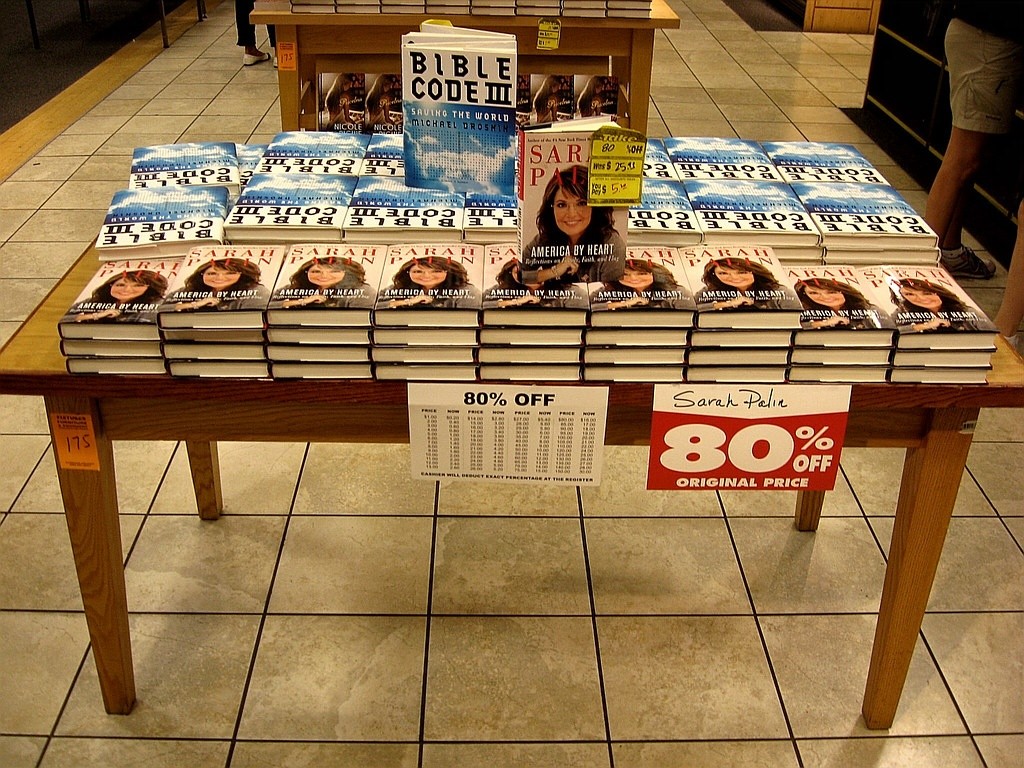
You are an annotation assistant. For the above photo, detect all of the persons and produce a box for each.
[74,268,162,324]
[525,163,624,278]
[883,281,983,330]
[385,253,480,308]
[920,0,1024,363]
[233,0,284,68]
[786,276,897,331]
[274,254,374,307]
[690,256,800,325]
[169,256,266,315]
[602,257,699,313]
[489,256,582,313]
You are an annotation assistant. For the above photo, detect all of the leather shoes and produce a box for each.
[243,53,272,66]
[273,57,278,68]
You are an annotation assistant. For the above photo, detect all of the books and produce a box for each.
[56,22,988,385]
[285,1,665,18]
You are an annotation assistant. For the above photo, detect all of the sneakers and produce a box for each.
[940,244,996,280]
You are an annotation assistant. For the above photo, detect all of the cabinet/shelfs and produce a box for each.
[860,0,1024,238]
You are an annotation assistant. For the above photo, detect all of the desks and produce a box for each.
[248,0,680,136]
[0,237,1024,730]
